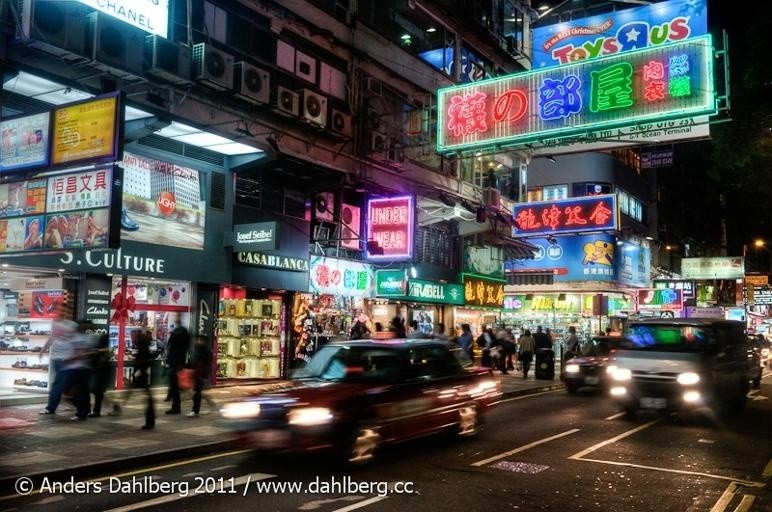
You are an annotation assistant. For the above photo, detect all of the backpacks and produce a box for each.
[476,334,487,349]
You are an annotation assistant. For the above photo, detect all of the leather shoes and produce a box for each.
[33,304,44,315]
[19,307,30,314]
[121,211,140,231]
[47,302,56,312]
[35,295,45,305]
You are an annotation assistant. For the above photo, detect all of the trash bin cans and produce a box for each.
[535,348,556,380]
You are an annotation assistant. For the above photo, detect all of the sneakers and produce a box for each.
[12,360,49,370]
[39,408,201,431]
[0,341,48,353]
[15,327,51,335]
[14,377,47,387]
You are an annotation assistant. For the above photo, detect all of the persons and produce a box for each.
[39,302,214,429]
[374,316,581,378]
[121,204,139,231]
[5,210,107,248]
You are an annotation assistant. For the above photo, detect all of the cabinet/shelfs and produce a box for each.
[0,313,57,395]
[107,323,144,342]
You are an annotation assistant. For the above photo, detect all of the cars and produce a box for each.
[219,337,504,463]
[558,318,772,424]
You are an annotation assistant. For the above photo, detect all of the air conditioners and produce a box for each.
[483,185,503,212]
[313,188,338,225]
[274,39,354,142]
[14,1,192,87]
[507,33,524,58]
[340,198,362,253]
[194,43,272,109]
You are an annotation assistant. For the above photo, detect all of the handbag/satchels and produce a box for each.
[521,350,533,363]
[175,367,196,391]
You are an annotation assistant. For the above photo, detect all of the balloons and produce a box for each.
[111,278,186,301]
